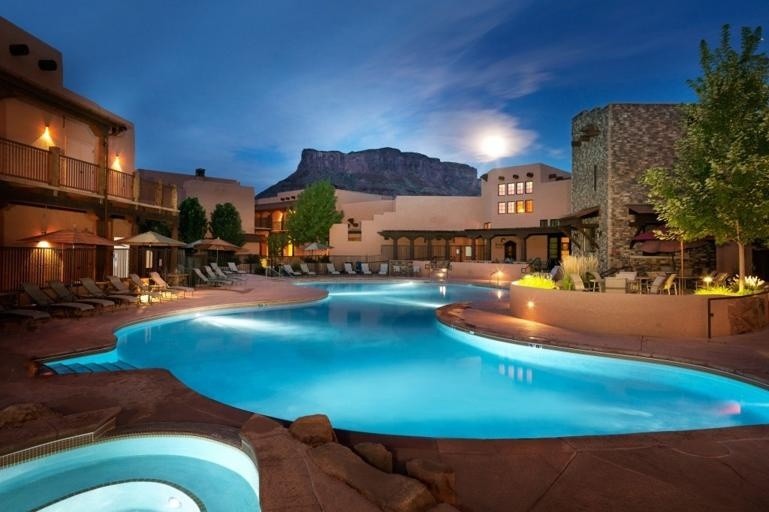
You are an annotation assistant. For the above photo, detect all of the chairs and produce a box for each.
[571,270,679,296]
[1,261,247,325]
[282,262,388,278]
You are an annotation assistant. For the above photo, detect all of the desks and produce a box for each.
[677,275,698,294]
